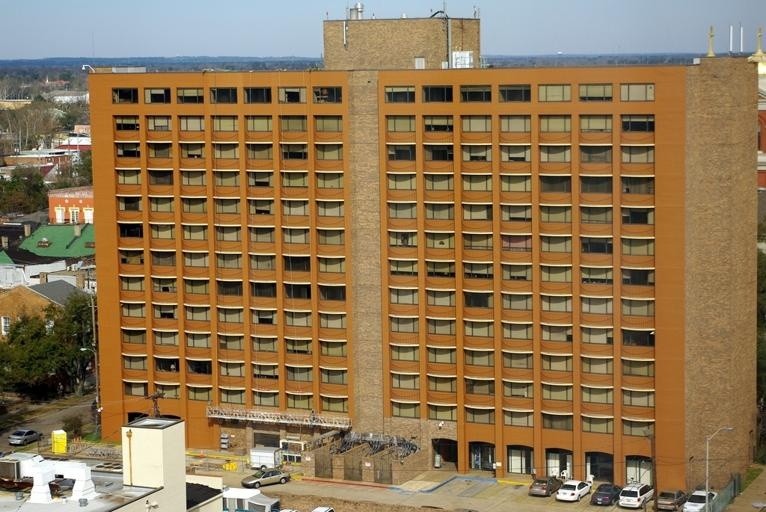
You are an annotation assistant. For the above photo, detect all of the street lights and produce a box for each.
[146,500,160,512]
[705,427,734,512]
[80,347,99,432]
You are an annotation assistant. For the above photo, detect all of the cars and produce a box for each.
[7,428,44,446]
[528,476,719,512]
[241,468,291,489]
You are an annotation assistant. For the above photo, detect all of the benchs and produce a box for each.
[0,476,33,490]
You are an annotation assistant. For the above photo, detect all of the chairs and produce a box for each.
[48,479,75,499]
